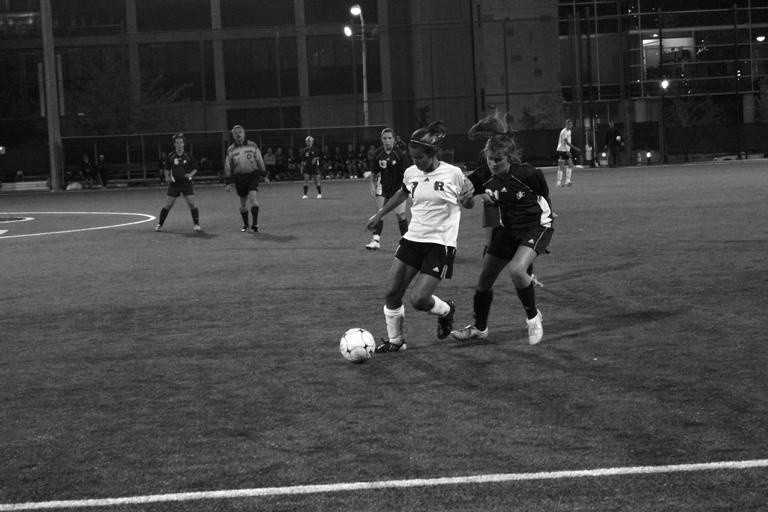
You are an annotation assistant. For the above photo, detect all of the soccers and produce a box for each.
[339,327,376,362]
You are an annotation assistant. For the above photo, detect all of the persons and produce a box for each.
[476,188,550,289]
[602,120,621,168]
[298,136,327,201]
[321,144,377,179]
[264,147,305,181]
[152,132,206,231]
[160,152,168,185]
[364,127,417,249]
[365,120,478,354]
[223,124,273,234]
[556,119,582,188]
[98,155,108,186]
[396,135,408,154]
[446,111,559,345]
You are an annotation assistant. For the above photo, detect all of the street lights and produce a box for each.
[755,34,767,44]
[659,79,671,163]
[350,3,369,128]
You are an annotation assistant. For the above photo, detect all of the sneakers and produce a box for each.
[241,225,249,232]
[251,224,260,232]
[194,226,201,230]
[565,180,572,186]
[302,195,308,199]
[155,224,161,232]
[450,325,489,341]
[374,337,407,353]
[365,239,380,248]
[317,194,322,198]
[526,309,543,345]
[556,182,564,187]
[437,300,456,339]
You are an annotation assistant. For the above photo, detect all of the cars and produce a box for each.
[713,151,767,161]
